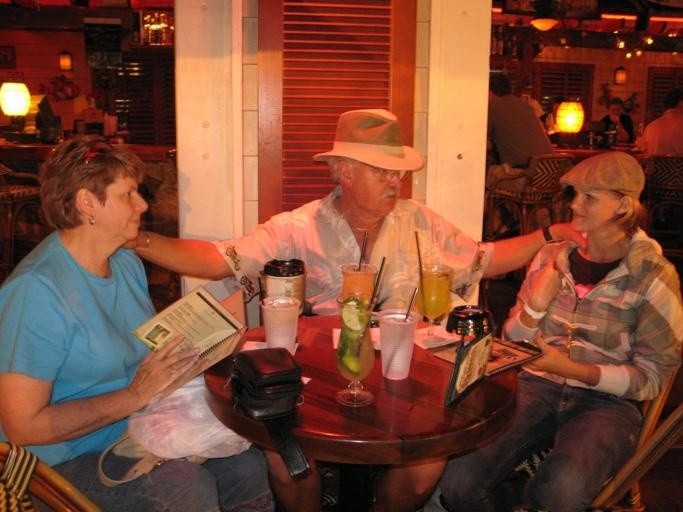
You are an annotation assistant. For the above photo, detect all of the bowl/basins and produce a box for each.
[445,305,494,336]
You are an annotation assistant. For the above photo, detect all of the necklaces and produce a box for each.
[337,204,379,232]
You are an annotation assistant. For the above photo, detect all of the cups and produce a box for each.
[337,264,375,303]
[377,308,419,381]
[413,263,452,345]
[259,296,302,355]
[333,293,375,406]
[259,258,306,306]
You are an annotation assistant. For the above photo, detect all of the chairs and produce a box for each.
[484,148,576,293]
[0,159,42,280]
[641,151,681,263]
[0,439,110,512]
[592,398,682,512]
[439,232,681,512]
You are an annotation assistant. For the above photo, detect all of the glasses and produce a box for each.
[335,157,409,182]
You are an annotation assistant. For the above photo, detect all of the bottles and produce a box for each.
[489,25,517,57]
[142,13,174,46]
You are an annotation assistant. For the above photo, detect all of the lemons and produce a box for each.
[340,296,361,331]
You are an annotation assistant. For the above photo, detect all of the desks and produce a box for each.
[203,299,530,512]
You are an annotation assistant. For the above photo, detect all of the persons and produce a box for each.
[420,150,681,512]
[121,109,587,511]
[639,87,682,228]
[602,97,635,142]
[0,135,280,512]
[486,71,555,239]
[540,96,568,133]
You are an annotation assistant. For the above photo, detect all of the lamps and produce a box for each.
[612,63,627,88]
[59,48,72,74]
[0,77,31,132]
[529,4,557,33]
[553,96,586,137]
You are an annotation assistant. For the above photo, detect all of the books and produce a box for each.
[129,286,250,381]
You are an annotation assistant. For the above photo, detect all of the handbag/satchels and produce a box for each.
[96,432,208,487]
[232,348,301,383]
[229,371,303,420]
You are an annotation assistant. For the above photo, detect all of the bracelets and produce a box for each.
[144,232,151,255]
[541,227,553,242]
[523,303,546,319]
[614,123,621,129]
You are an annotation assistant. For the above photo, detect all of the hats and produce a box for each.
[560,152,646,198]
[312,109,423,171]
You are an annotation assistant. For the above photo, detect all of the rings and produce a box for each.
[169,363,177,373]
